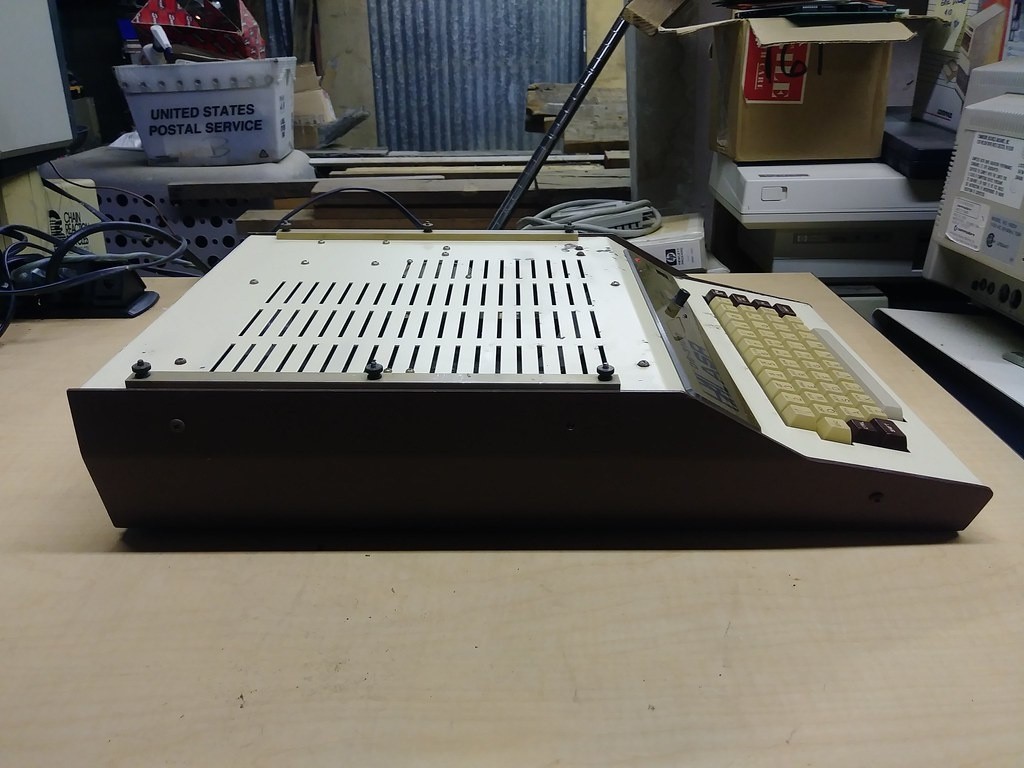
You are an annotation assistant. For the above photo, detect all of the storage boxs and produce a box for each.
[660,1,953,162]
[295,62,327,125]
[114,58,299,167]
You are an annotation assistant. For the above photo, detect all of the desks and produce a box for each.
[872,306,1024,458]
[39,100,717,279]
[0,272,1024,767]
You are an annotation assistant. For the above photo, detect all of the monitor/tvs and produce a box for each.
[922,55,1024,368]
